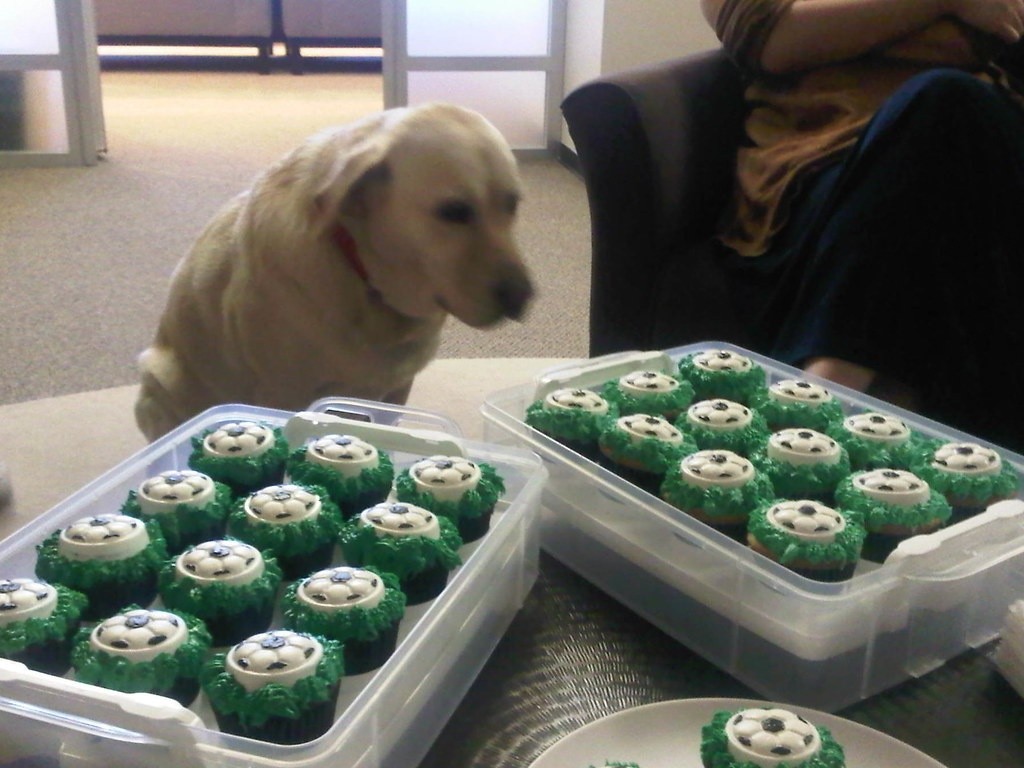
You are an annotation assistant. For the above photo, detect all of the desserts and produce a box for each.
[0,349,1024,768]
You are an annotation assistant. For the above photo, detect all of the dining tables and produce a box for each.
[0,358,1024,768]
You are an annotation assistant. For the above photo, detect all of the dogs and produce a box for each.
[133,101,539,446]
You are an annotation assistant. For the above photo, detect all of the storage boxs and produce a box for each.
[1,397,546,768]
[479,340,1024,713]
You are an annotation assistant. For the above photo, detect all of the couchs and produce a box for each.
[561,50,742,359]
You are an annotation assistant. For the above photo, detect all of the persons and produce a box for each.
[703,0,1024,454]
